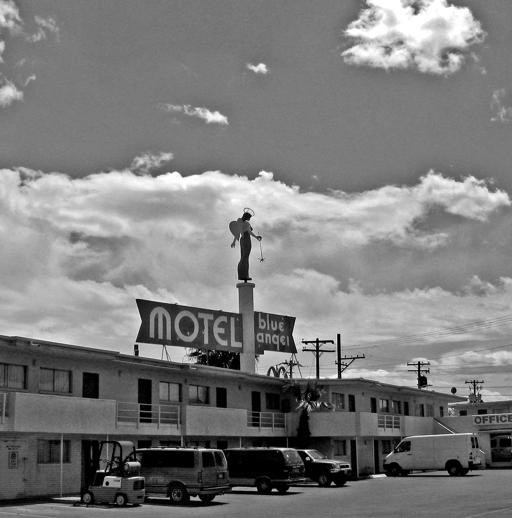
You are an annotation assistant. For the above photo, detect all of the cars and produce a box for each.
[297,448,352,487]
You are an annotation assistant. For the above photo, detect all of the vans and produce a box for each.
[123,446,310,505]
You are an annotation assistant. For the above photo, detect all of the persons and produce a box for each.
[231,213,262,280]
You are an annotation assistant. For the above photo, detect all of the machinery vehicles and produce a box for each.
[50,441,145,507]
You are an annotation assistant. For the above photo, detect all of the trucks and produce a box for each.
[383,433,481,477]
